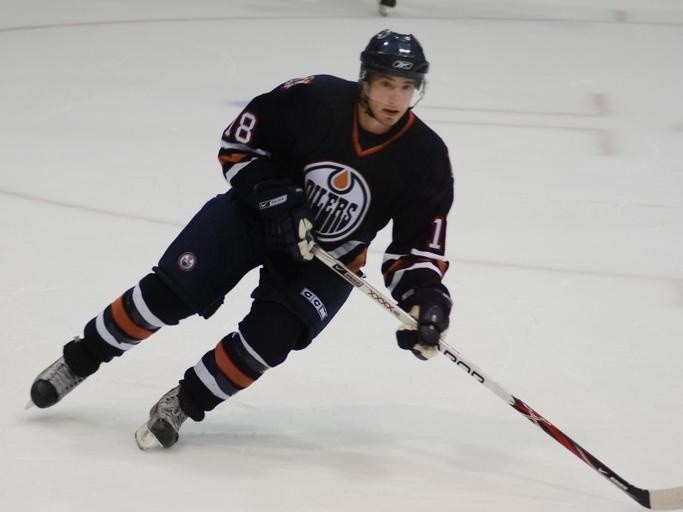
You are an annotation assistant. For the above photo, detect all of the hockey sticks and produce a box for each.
[307,231,683,511]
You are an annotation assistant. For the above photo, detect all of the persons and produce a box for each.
[30,28,453,451]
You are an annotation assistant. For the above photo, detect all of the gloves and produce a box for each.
[396,282,453,362]
[247,182,316,269]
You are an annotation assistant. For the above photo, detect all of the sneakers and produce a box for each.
[31,355,87,408]
[149,383,187,449]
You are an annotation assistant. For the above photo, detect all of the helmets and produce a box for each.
[360,29,429,108]
